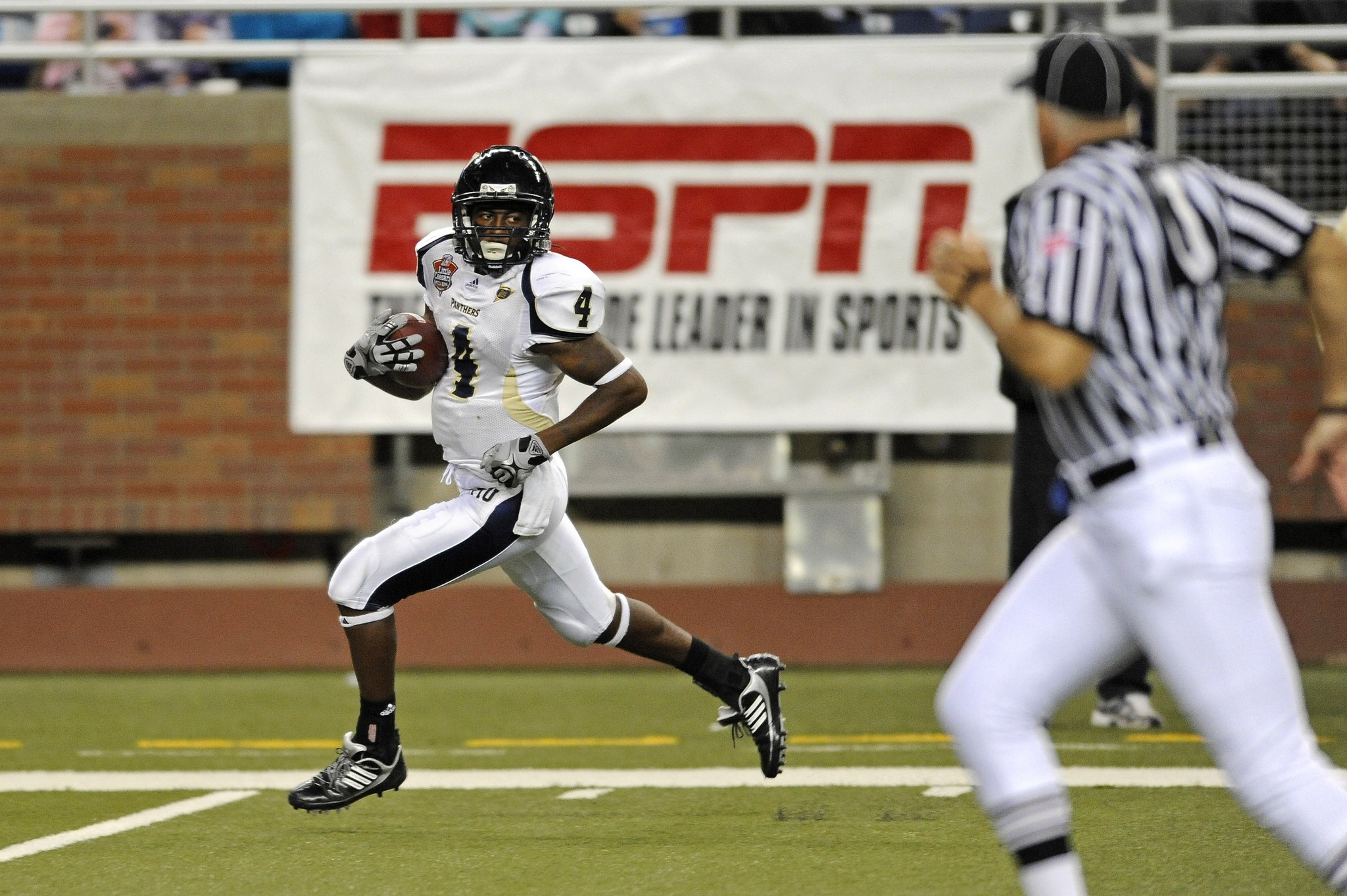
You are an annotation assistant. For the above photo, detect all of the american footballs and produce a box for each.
[385,313,449,387]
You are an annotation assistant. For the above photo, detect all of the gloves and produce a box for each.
[480,434,552,489]
[344,306,425,380]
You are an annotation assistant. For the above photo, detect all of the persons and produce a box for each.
[930,29,1347,895]
[287,146,786,813]
[996,193,1165,728]
[0,1,1347,136]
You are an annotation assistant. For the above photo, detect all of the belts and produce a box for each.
[1086,432,1222,491]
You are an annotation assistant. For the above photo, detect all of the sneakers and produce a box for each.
[716,652,795,778]
[1090,692,1164,732]
[287,732,407,815]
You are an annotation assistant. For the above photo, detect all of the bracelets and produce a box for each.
[1319,405,1346,414]
[956,269,991,306]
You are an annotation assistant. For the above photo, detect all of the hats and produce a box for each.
[1009,34,1139,117]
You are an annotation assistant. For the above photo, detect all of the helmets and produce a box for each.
[450,145,555,279]
